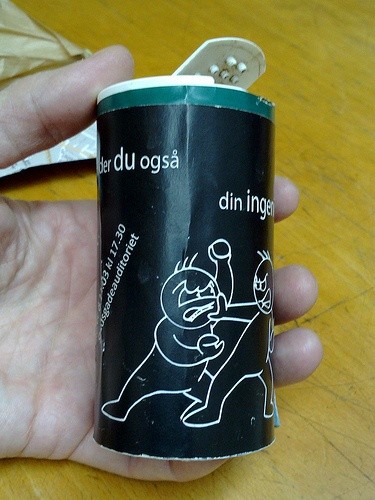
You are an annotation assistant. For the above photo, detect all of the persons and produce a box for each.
[0,44,324,484]
